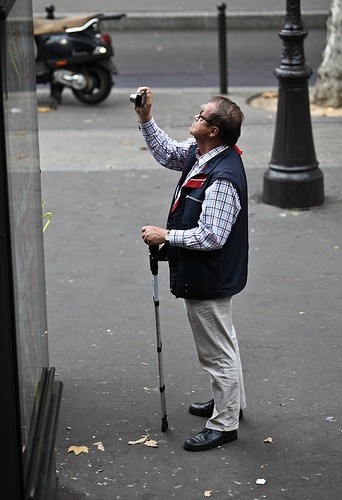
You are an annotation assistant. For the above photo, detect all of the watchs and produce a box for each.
[165,229,171,246]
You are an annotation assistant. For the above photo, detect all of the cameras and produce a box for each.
[130,90,146,107]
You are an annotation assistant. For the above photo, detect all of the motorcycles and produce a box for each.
[31,11,126,110]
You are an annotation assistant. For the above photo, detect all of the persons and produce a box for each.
[135,86,249,451]
